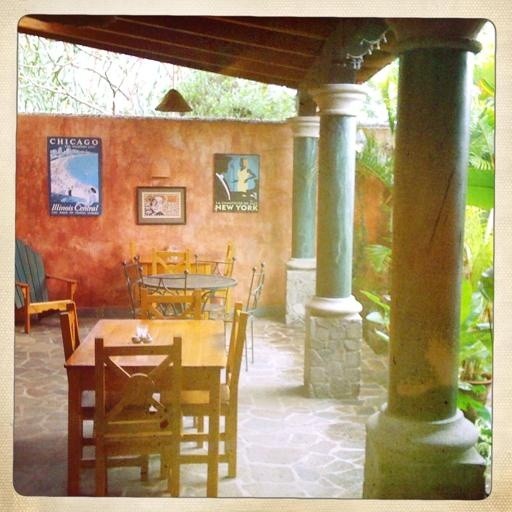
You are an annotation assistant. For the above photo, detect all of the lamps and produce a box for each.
[153,61,195,113]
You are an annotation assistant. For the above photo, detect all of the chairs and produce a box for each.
[160,301,248,480]
[14,238,79,336]
[140,287,201,320]
[61,301,148,482]
[122,243,265,372]
[94,337,182,497]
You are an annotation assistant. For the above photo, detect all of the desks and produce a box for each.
[64,319,226,497]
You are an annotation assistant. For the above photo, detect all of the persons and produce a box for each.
[145,194,168,216]
[214,155,256,203]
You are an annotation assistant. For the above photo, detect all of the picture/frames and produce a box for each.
[45,135,104,217]
[137,187,186,226]
[213,151,262,213]
